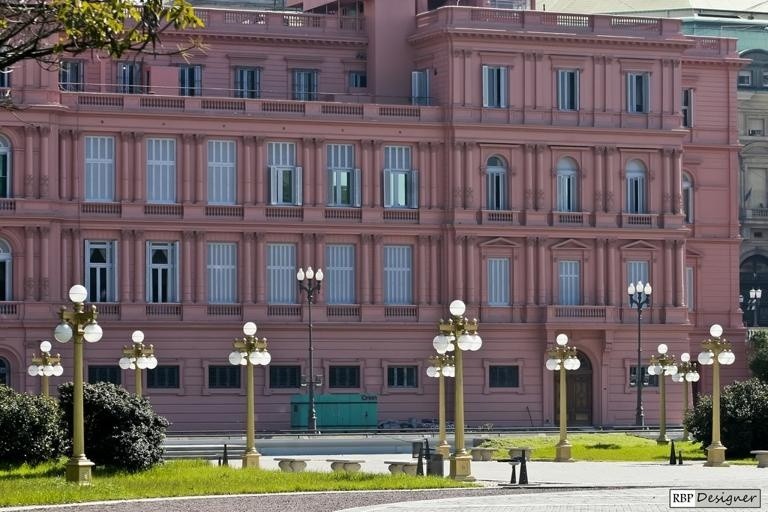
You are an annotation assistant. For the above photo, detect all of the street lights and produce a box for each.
[544,333,583,462]
[748,285,762,329]
[627,280,652,432]
[27,340,64,401]
[228,321,272,471]
[53,283,104,487]
[296,265,324,436]
[118,329,160,400]
[425,298,484,483]
[647,323,736,467]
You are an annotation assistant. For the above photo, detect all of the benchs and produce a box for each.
[274,457,417,477]
[471,446,535,462]
